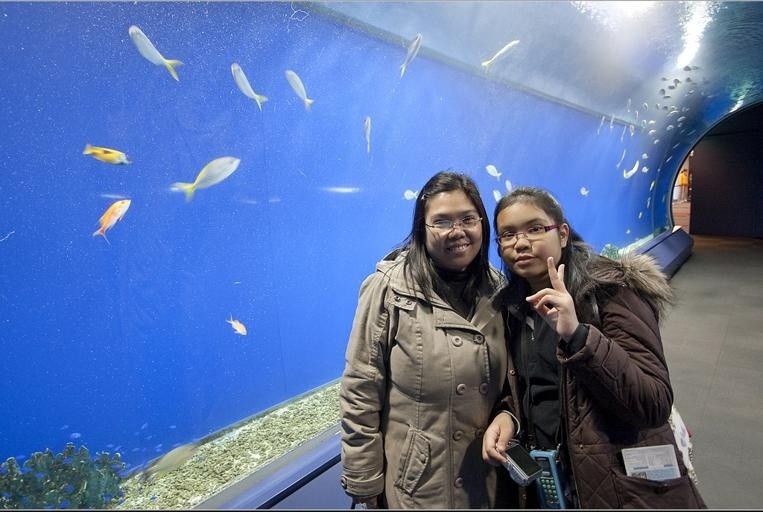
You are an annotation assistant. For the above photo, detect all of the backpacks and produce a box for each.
[665,402,699,487]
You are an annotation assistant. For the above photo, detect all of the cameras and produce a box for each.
[496,439,543,487]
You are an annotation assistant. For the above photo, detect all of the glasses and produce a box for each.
[494,223,563,248]
[421,217,484,235]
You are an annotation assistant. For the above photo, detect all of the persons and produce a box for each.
[340,169,517,512]
[677,170,688,204]
[482,187,709,512]
